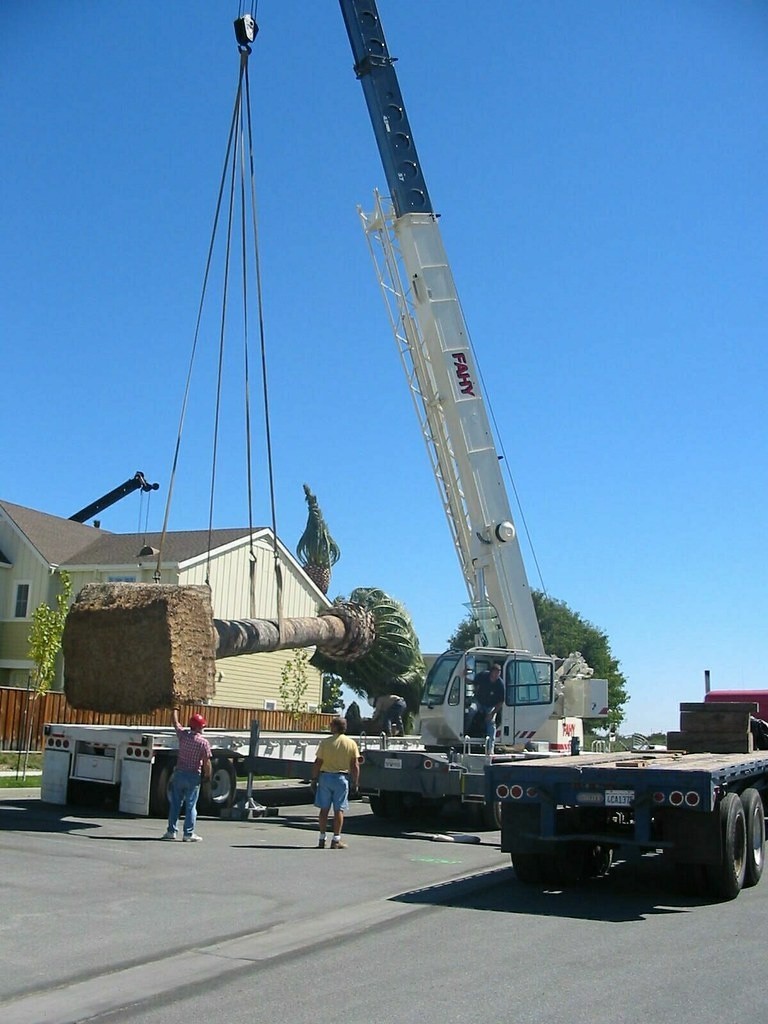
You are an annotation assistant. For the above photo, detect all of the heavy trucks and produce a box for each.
[482,668,768,902]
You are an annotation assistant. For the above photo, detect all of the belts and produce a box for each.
[321,770,348,775]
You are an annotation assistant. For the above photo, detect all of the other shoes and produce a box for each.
[488,750,494,755]
[465,735,470,740]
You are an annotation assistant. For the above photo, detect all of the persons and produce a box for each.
[163,698,213,842]
[310,718,360,850]
[368,695,406,737]
[464,664,505,754]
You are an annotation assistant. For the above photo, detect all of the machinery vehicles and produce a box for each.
[150,0,609,831]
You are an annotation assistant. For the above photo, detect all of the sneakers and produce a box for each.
[319,838,326,848]
[163,832,176,839]
[183,833,203,842]
[331,840,347,849]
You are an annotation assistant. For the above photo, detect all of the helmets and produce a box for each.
[189,714,206,728]
